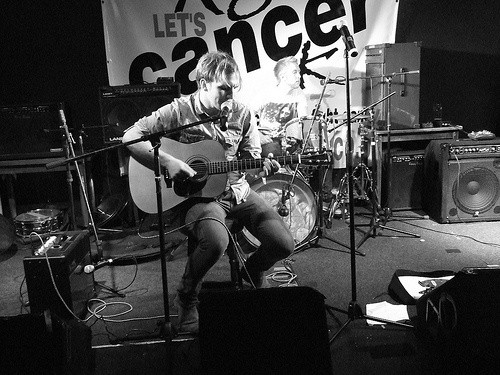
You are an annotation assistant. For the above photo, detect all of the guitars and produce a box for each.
[127,135,333,216]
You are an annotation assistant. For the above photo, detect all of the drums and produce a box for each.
[283,116,331,154]
[326,114,376,173]
[234,172,319,254]
[14,205,66,239]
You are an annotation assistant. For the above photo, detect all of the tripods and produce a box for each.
[292,40,417,347]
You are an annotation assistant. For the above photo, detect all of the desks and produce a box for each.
[0,151,98,227]
[367,123,463,211]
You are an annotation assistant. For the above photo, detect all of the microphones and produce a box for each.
[340,26,358,57]
[279,186,289,216]
[220,101,232,132]
[84,258,116,273]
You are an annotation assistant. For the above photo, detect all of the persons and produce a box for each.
[123,52,294,333]
[252,56,347,215]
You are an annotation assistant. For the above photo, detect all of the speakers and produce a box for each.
[198,285,333,375]
[98,82,181,144]
[410,268,500,364]
[0,314,96,375]
[364,40,422,129]
[23,229,97,321]
[0,101,67,160]
[422,137,500,224]
[382,148,426,210]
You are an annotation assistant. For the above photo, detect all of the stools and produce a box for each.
[178,219,244,295]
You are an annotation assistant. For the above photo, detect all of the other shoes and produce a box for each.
[323,202,348,214]
[240,265,272,288]
[178,303,199,333]
